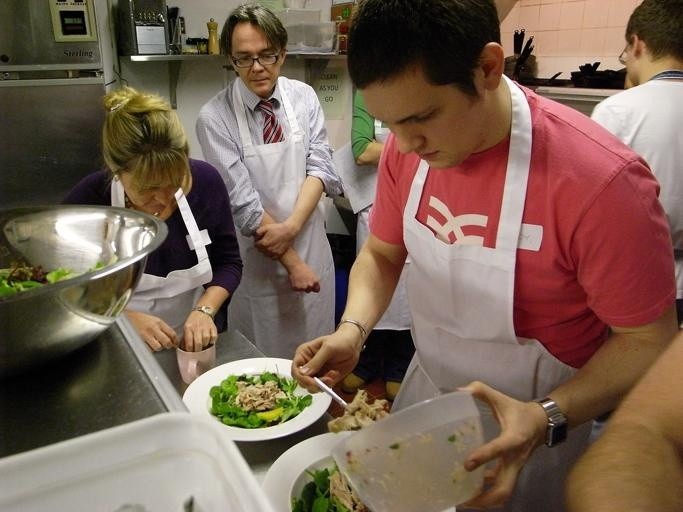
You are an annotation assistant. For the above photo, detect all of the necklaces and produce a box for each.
[124,195,161,219]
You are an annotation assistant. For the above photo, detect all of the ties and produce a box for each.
[256,99,285,144]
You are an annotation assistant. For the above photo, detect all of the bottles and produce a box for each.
[207,18,220,55]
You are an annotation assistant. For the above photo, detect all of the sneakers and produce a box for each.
[341,373,365,394]
[385,381,400,401]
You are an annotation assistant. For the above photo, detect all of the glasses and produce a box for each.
[229,49,280,68]
[618,42,630,65]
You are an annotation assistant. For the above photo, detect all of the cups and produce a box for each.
[176,334,215,384]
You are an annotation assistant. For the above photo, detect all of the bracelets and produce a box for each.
[338,319,370,351]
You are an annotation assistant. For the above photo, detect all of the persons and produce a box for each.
[341,85,411,403]
[590,2,683,439]
[563,329,683,512]
[60,86,242,354]
[290,0,679,512]
[193,2,338,359]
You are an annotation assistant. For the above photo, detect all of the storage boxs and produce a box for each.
[282,21,334,52]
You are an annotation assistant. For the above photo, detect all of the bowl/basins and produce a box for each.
[1,203,171,375]
[331,392,485,512]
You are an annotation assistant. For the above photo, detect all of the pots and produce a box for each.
[504,29,626,89]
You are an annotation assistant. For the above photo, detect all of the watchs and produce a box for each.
[539,396,570,448]
[192,305,217,322]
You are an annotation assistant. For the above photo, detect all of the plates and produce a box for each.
[262,431,456,512]
[0,412,274,512]
[182,358,333,442]
